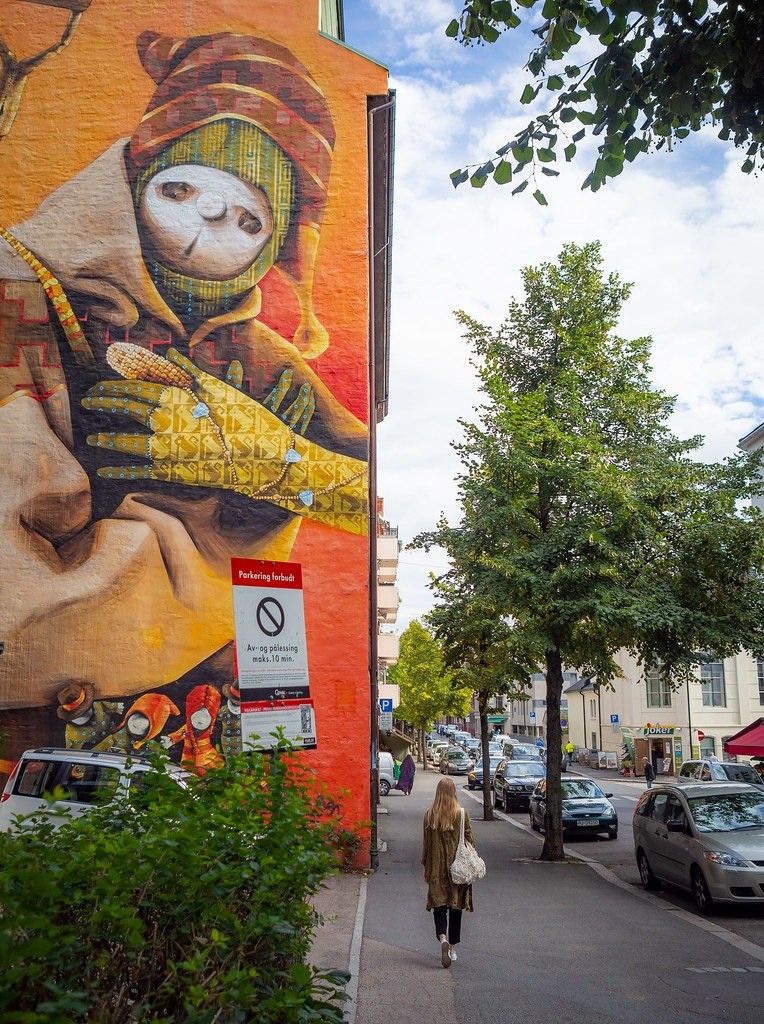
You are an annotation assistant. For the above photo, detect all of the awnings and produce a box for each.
[487,718,506,723]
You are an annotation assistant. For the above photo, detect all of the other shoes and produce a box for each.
[441,939,451,968]
[450,949,456,961]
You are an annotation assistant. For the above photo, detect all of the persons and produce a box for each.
[709,752,720,762]
[496,729,501,734]
[440,725,449,738]
[396,755,416,796]
[642,757,655,788]
[536,739,544,747]
[491,729,494,738]
[421,778,476,968]
[565,741,575,766]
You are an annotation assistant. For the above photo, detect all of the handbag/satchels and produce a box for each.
[451,808,487,884]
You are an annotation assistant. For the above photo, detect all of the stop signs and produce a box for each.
[698,730,705,741]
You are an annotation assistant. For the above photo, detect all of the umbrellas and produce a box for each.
[723,716,764,763]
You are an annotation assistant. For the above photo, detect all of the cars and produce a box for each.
[529,774,618,840]
[633,781,762,907]
[467,756,509,791]
[492,735,569,773]
[439,748,475,775]
[475,741,502,757]
[425,725,481,767]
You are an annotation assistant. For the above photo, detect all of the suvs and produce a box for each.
[0,749,269,853]
[495,760,546,813]
[678,759,763,789]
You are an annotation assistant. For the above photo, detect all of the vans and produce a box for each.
[379,752,398,796]
